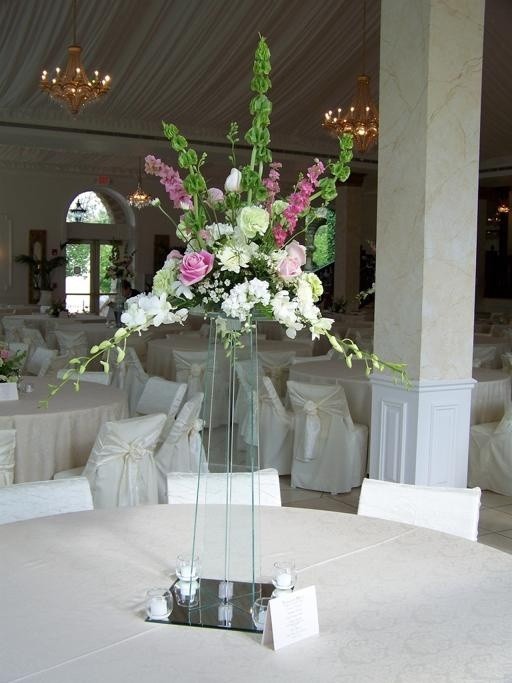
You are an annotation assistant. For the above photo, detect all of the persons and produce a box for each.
[108,280,141,327]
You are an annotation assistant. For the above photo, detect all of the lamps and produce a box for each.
[321,74,379,160]
[126,189,151,211]
[38,44,111,120]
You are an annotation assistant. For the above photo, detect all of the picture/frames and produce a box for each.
[28,229,47,304]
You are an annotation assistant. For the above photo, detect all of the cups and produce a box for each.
[177,555,202,581]
[251,598,274,630]
[144,586,175,621]
[174,580,201,607]
[271,561,299,590]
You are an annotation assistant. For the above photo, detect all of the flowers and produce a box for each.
[38,36,409,410]
[104,236,139,282]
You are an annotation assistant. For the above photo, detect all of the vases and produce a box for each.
[106,277,131,319]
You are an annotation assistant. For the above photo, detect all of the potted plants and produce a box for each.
[15,254,72,305]
